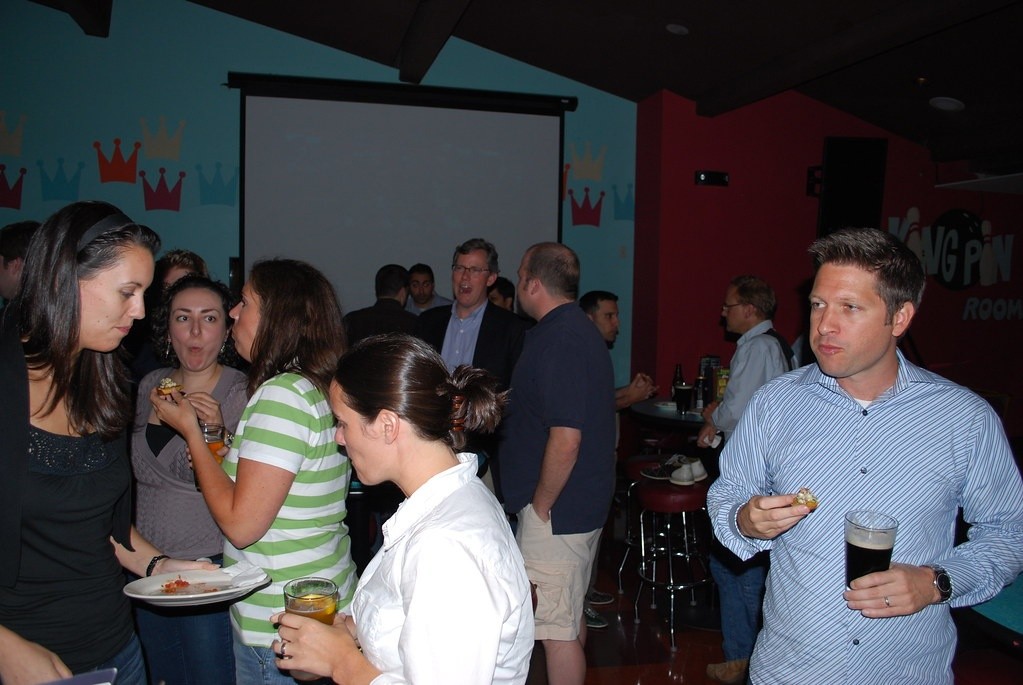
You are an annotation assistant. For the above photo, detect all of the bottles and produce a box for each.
[694,376,710,410]
[671,362,686,402]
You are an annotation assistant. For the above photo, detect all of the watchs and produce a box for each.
[919,563,953,603]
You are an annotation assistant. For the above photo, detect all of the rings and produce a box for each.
[883,597,891,607]
[280,643,287,655]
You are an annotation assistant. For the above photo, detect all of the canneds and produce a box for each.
[694,377,706,409]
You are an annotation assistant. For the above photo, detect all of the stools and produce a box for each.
[612,443,705,610]
[631,471,715,653]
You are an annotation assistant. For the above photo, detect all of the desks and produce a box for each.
[618,400,723,457]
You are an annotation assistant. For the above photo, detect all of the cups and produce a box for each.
[703,434,721,449]
[283,576,339,680]
[844,508,900,592]
[675,384,694,412]
[193,422,225,493]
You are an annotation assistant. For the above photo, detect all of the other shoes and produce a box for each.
[707,658,750,684]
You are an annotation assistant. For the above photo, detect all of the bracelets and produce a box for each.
[224,430,234,447]
[357,646,363,653]
[146,555,171,577]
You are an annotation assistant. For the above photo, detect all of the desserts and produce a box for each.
[158,377,184,395]
[789,487,817,511]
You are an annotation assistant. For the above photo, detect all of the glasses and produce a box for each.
[452,265,489,273]
[723,304,741,310]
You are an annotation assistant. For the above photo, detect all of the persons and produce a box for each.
[576,290,662,627]
[273,333,538,685]
[697,274,800,683]
[705,229,1023,685]
[0,198,162,685]
[339,238,537,582]
[498,241,618,685]
[0,220,43,319]
[127,248,252,685]
[149,260,361,685]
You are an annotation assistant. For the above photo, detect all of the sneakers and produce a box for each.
[585,588,615,604]
[583,605,608,627]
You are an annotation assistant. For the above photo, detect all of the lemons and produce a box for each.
[295,594,335,614]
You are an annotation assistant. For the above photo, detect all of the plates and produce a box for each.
[123,567,272,607]
